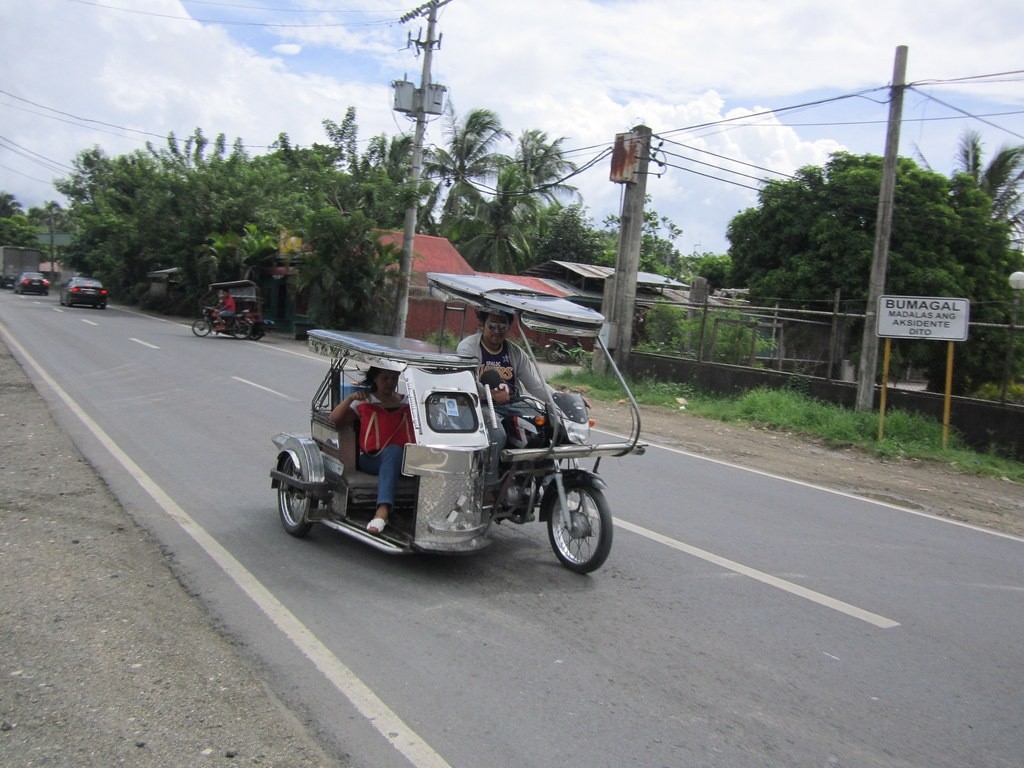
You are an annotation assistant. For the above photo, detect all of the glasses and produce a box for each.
[485,319,511,334]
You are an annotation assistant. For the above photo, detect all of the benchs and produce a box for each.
[321,423,416,514]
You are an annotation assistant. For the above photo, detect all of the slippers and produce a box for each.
[367,517,389,534]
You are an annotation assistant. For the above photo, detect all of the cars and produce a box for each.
[14,272,49,296]
[59,277,108,309]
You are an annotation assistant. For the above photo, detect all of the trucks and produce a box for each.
[0,246,41,287]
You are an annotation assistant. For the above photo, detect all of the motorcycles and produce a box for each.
[191,280,275,340]
[271,272,651,574]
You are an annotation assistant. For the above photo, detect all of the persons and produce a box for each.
[328,366,417,536]
[214,289,237,331]
[456,311,592,502]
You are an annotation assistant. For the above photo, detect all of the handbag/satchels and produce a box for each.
[356,401,417,457]
